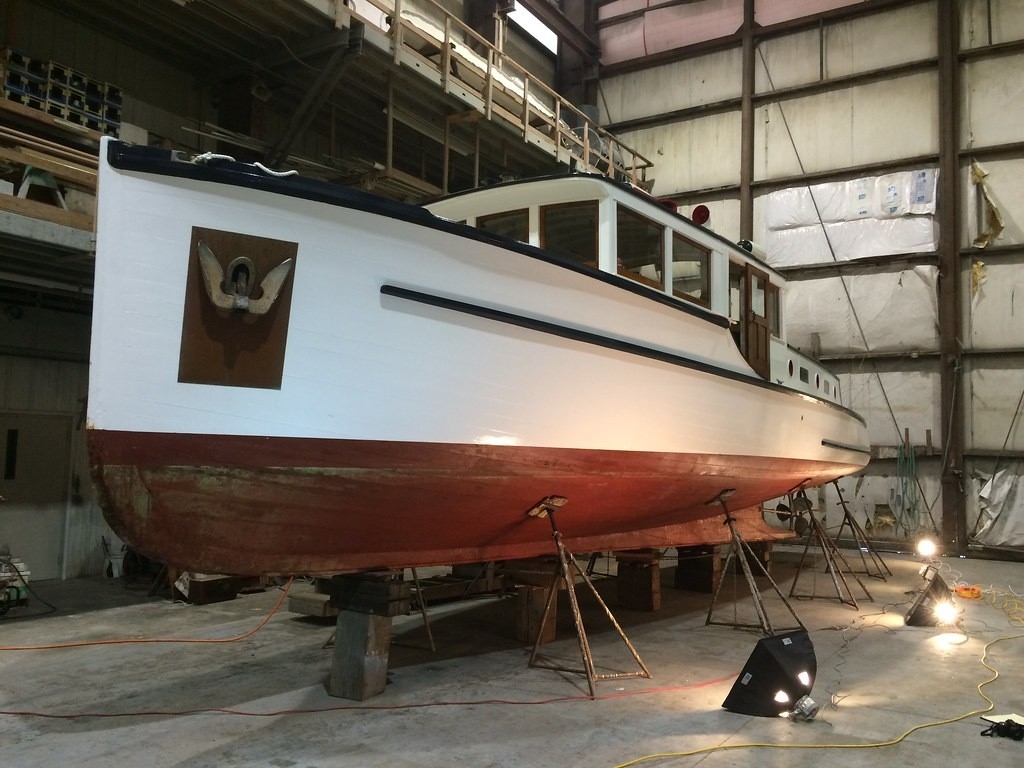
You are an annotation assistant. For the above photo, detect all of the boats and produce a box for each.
[79,132,871,578]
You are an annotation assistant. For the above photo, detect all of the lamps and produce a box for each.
[905,572,960,627]
[721,628,820,723]
